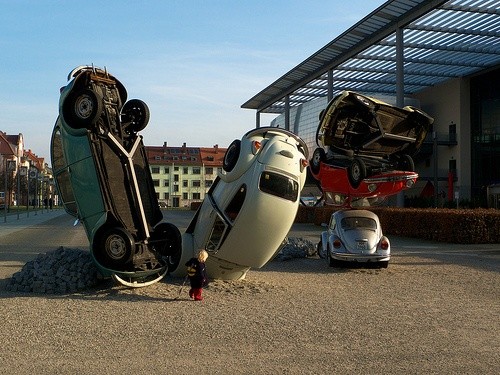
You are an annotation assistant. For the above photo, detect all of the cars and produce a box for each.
[169,125,309,282]
[317,208,391,268]
[316,91,435,157]
[49,63,182,288]
[309,148,419,209]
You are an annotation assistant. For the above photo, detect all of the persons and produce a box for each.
[44,197,48,209]
[420,178,434,197]
[49,198,53,209]
[185,249,209,300]
